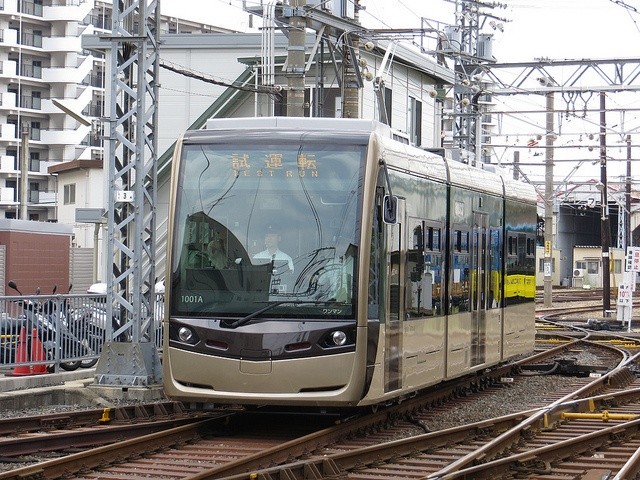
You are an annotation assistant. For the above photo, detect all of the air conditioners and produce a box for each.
[573,270,584,277]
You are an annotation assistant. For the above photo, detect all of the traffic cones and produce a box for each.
[12,326,31,376]
[31,328,50,375]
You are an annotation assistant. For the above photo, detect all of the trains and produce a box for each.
[162,117,537,411]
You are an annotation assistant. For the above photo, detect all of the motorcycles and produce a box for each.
[0,286,84,371]
[55,284,98,368]
[0,281,61,372]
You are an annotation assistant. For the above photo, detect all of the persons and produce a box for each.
[302,232,354,302]
[252,223,294,278]
